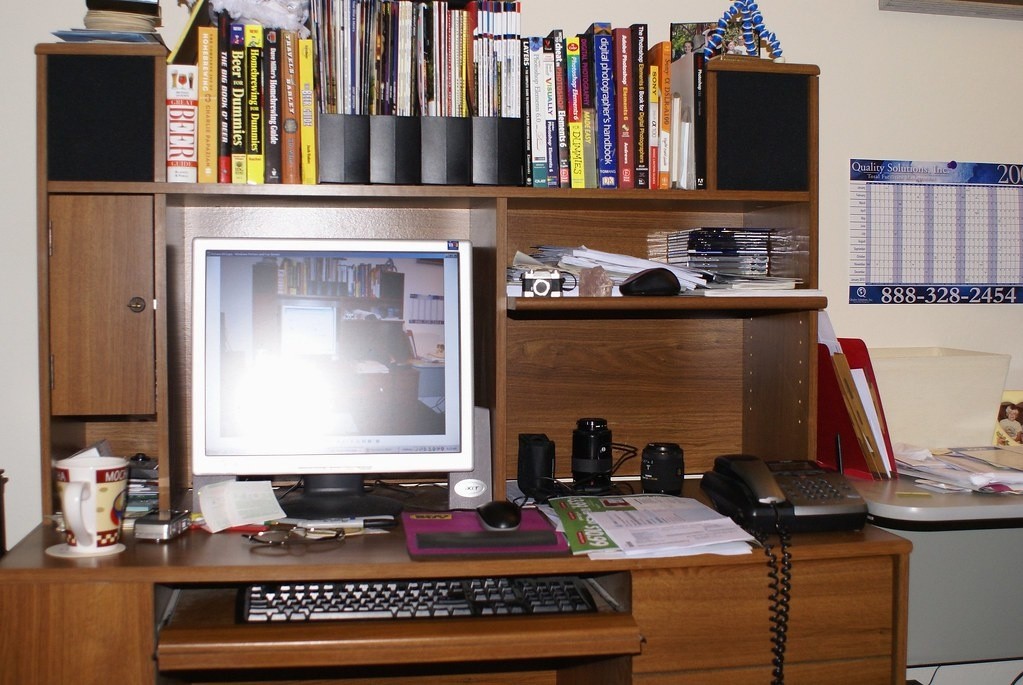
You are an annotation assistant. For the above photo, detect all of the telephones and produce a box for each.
[699,453,867,539]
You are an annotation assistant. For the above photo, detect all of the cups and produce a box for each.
[52,456,130,555]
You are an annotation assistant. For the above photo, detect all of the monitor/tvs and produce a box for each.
[189,238,474,524]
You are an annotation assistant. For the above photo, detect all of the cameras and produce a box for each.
[521,269,564,297]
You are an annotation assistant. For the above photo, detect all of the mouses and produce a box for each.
[618,268,683,295]
[483,499,522,531]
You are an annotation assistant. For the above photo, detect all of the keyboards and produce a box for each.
[233,579,597,624]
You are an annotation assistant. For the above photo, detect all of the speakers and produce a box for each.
[434,407,492,510]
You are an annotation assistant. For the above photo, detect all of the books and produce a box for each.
[891,446,1022,495]
[121,478,162,531]
[279,255,381,298]
[163,0,709,190]
[506,226,820,298]
[812,309,899,482]
[417,351,445,363]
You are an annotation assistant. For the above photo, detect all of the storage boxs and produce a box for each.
[868,343,1012,449]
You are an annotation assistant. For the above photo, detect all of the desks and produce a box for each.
[1,478,914,685]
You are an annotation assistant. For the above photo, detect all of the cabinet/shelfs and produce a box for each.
[847,479,1023,684]
[33,42,829,523]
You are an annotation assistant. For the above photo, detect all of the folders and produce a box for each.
[817,311,897,482]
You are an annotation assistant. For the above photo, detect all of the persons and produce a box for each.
[680,40,694,59]
[1000,405,1023,444]
